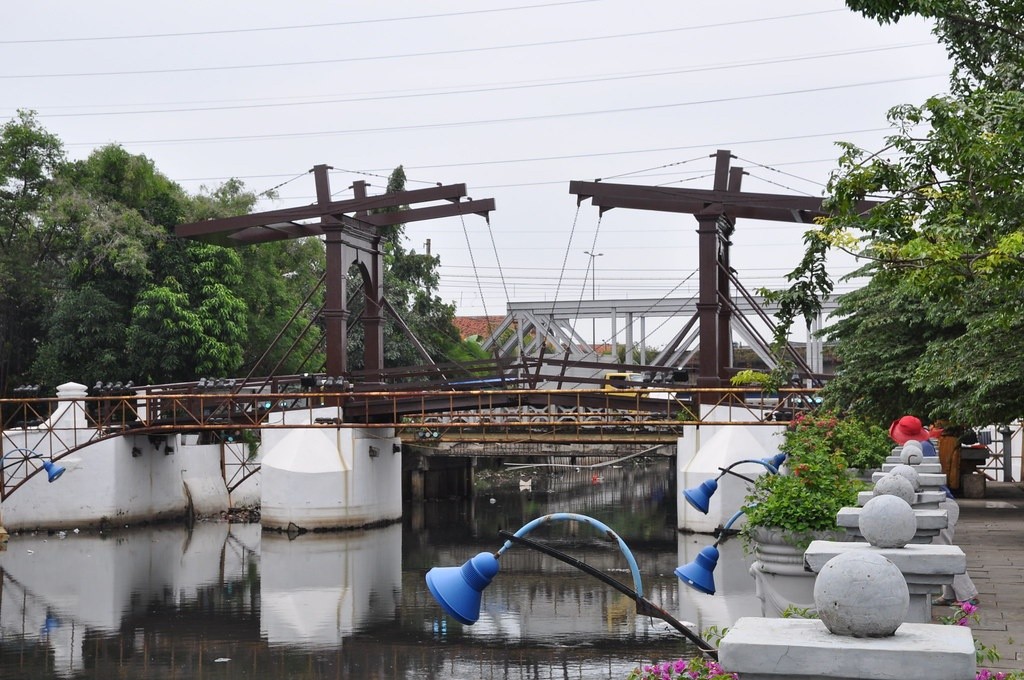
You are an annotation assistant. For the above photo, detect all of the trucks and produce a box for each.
[518,353,649,400]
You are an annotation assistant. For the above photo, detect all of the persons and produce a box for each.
[887,414,953,498]
[928,416,949,439]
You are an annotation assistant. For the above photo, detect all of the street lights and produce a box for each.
[584,251,603,351]
[602,340,606,351]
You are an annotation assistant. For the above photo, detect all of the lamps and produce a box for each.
[765,412,792,421]
[425,513,643,625]
[301,373,316,385]
[792,372,800,381]
[0,447,66,483]
[93,381,134,391]
[762,450,789,474]
[674,502,762,595]
[643,371,663,382]
[325,376,345,386]
[198,377,236,390]
[14,384,39,395]
[680,459,779,516]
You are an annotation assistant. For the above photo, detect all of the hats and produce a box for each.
[888,415,930,446]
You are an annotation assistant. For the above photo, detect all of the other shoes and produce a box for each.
[931,596,956,606]
[952,598,980,606]
